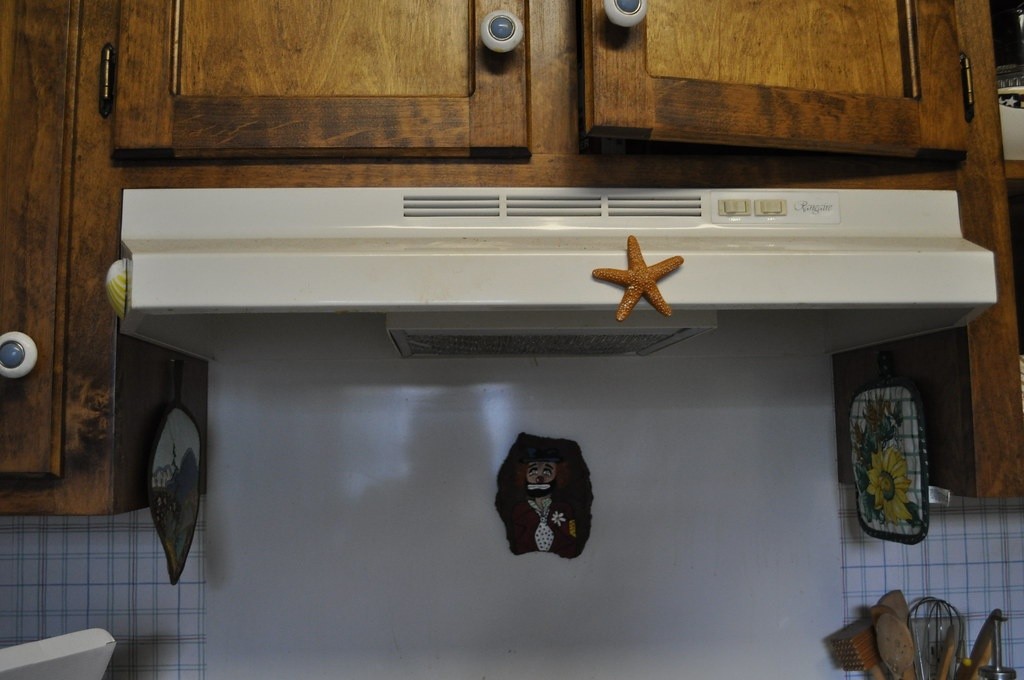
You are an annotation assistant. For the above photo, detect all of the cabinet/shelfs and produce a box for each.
[0,0,1024,517]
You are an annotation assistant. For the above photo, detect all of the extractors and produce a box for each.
[123,188,998,358]
